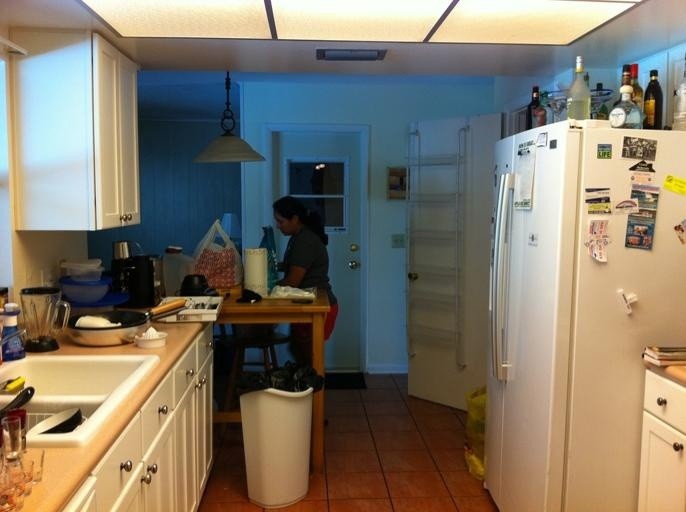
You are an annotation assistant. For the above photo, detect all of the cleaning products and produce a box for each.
[0,302,26,360]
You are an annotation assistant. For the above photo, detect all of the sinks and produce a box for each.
[1,355,159,399]
[1,399,116,445]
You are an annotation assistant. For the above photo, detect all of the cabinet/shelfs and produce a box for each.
[59,322,214,512]
[10,28,141,231]
[635,369,686,511]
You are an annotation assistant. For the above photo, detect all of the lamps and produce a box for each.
[193,69,266,163]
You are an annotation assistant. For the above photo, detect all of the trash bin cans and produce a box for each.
[237,361,323,509]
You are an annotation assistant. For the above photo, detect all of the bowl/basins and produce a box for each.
[26,408,82,436]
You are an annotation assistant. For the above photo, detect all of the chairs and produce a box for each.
[219,323,289,433]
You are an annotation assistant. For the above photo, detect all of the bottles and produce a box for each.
[524,53,685,130]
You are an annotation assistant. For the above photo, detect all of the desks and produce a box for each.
[216,288,331,474]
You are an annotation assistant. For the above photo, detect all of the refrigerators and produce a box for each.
[482,119,685,512]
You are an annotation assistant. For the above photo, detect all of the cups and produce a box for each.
[19,286,70,352]
[0,416,45,512]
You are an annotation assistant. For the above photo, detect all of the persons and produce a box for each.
[271,195,339,366]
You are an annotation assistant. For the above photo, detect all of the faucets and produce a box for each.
[0,329,28,346]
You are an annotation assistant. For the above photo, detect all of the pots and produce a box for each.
[63,299,188,347]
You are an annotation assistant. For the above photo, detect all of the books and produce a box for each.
[641,341,686,368]
